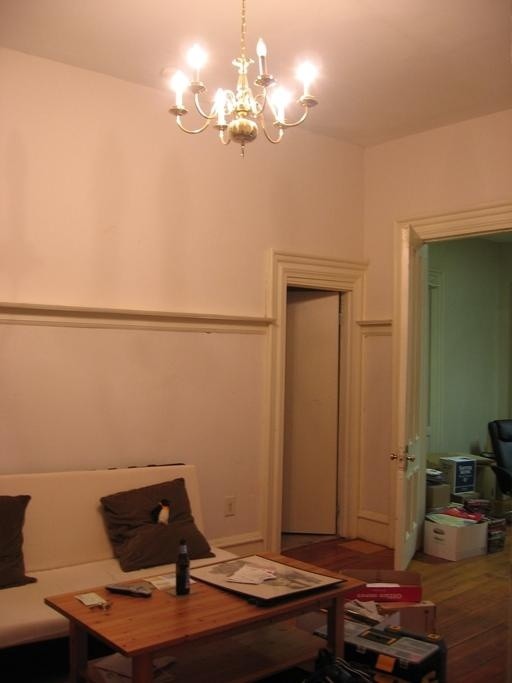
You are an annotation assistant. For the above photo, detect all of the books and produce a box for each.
[94,652,176,678]
[98,669,174,682]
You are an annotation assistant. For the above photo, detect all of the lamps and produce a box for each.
[168,1,318,159]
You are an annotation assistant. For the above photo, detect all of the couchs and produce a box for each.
[0,463,241,651]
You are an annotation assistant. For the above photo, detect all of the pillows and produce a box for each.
[0,494,37,590]
[99,477,216,572]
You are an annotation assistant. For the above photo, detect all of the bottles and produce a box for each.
[174,538,192,596]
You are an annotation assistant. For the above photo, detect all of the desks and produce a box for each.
[44,552,366,683]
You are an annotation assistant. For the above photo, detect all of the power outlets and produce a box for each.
[426,425,433,439]
[223,496,239,519]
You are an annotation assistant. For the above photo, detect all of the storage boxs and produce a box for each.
[376,595,438,633]
[439,456,476,495]
[423,519,488,562]
[87,651,177,683]
[426,484,450,512]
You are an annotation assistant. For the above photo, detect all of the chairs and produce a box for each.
[488,420,512,496]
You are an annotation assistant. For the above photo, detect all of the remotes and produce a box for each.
[105,584,152,598]
